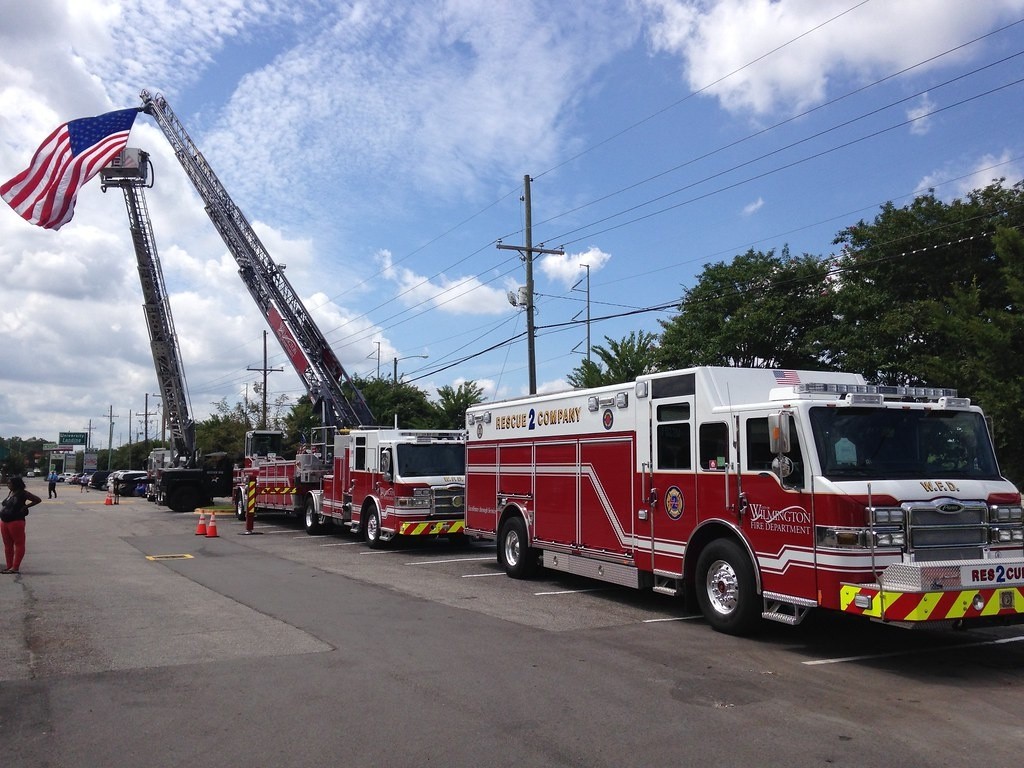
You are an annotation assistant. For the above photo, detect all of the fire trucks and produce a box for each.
[232,424,478,545]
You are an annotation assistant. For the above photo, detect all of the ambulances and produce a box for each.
[463,365,1024,636]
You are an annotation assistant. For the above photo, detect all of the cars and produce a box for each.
[59,469,149,498]
[26,472,34,477]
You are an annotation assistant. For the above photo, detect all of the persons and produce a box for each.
[47,471,57,498]
[0,476,42,574]
[81,473,90,493]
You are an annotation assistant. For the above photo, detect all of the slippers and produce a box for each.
[0,568,22,574]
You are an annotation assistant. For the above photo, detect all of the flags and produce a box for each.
[0,107,139,231]
[297,434,306,454]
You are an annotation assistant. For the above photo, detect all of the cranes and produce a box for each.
[99,143,234,512]
